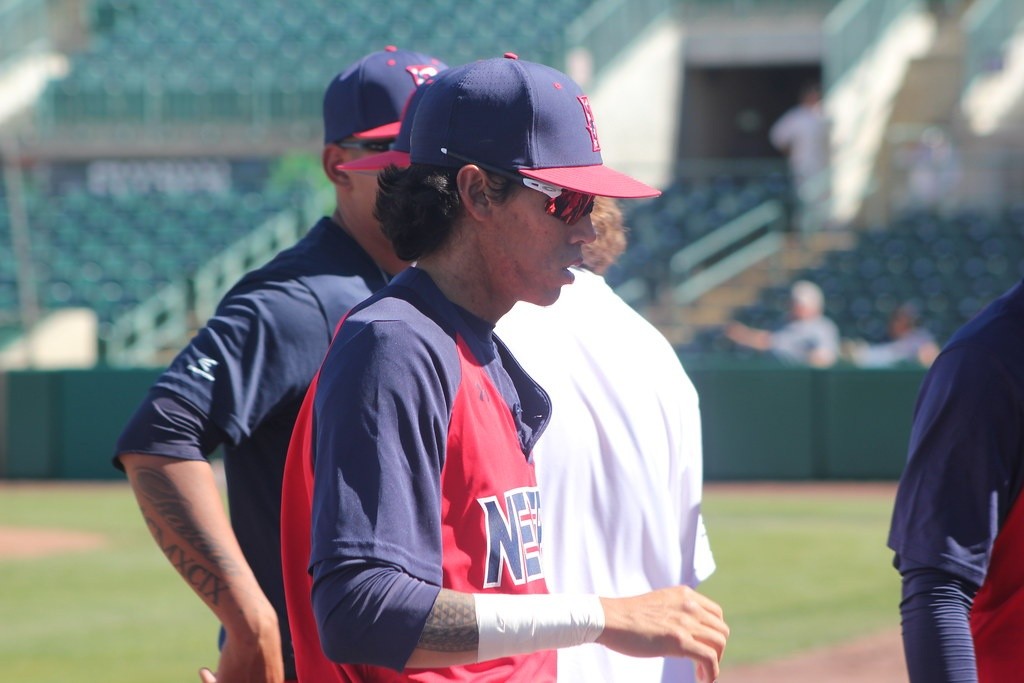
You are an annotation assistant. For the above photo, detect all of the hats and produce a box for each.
[409,53,663,198]
[323,45,451,145]
[336,70,450,171]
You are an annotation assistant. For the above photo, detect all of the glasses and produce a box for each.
[338,139,393,151]
[440,147,596,225]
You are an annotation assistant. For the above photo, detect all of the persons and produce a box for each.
[722,279,841,369]
[282,53,729,683]
[886,280,1024,683]
[770,85,825,221]
[112,46,450,682]
[498,193,715,683]
[844,298,939,371]
